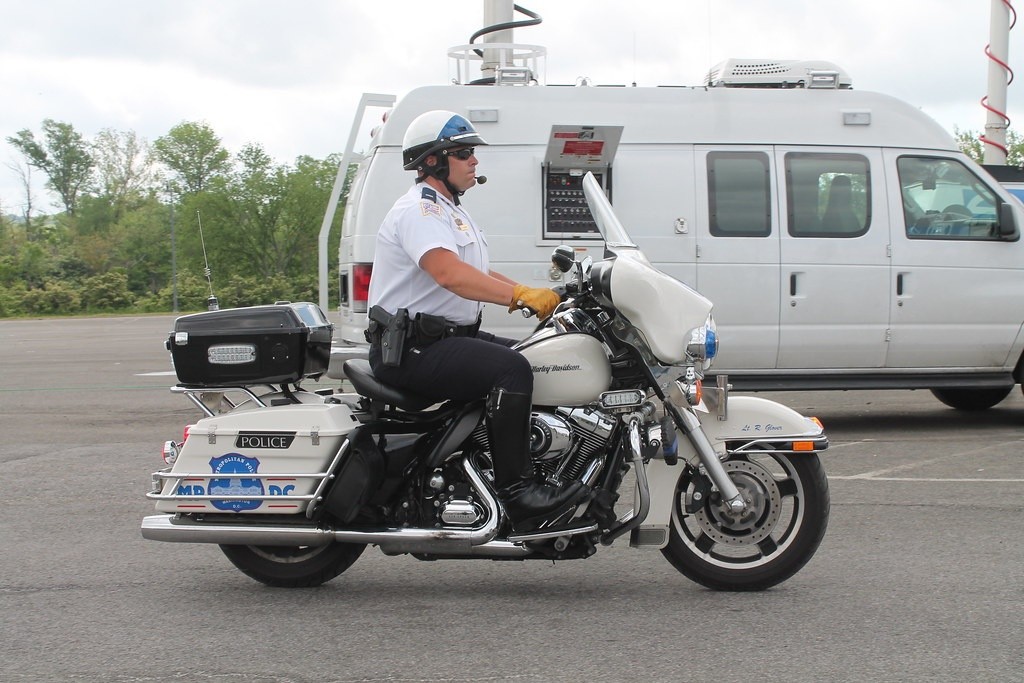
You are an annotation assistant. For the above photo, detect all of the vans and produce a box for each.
[325,43,1024,415]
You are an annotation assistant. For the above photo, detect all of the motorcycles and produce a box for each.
[138,168,835,594]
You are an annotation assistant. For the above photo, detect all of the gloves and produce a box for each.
[508,283,560,321]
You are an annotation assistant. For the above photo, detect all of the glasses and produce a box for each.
[434,148,477,160]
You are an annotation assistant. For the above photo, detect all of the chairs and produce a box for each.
[821,175,861,233]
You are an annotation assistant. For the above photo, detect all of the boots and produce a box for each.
[485,387,591,534]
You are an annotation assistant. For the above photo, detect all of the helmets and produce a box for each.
[403,110,489,170]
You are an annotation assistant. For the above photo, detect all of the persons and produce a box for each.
[365,109,584,533]
